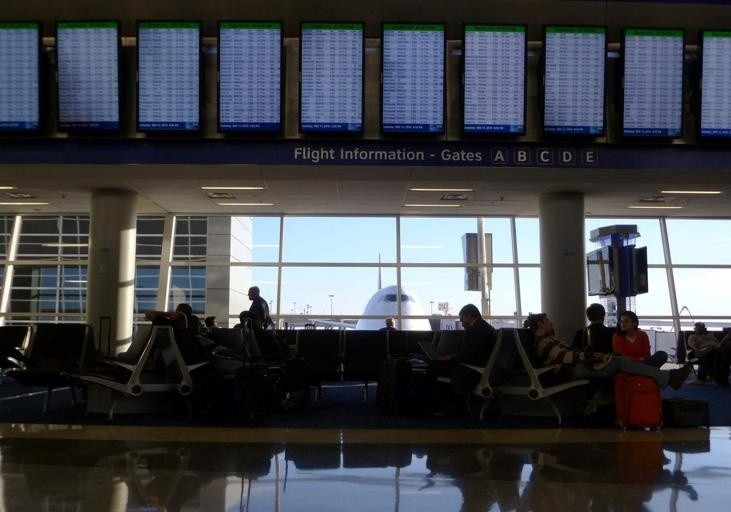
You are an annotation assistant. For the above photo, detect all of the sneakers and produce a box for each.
[667,363,694,391]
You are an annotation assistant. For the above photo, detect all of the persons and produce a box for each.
[686,331,731,384]
[75,286,270,391]
[571,304,614,353]
[524,314,693,390]
[612,312,651,423]
[426,304,496,379]
[377,318,397,330]
[688,323,719,362]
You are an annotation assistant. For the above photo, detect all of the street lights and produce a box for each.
[329,295,335,331]
[429,301,435,316]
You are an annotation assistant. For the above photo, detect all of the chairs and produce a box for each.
[0,322,730,437]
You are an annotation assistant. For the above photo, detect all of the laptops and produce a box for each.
[417,341,451,361]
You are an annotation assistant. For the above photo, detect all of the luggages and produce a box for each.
[613,369,664,433]
[375,353,439,429]
[663,397,710,429]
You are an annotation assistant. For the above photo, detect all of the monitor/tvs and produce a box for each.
[636,246,647,294]
[587,246,613,295]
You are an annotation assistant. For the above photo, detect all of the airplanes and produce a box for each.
[270,251,528,331]
